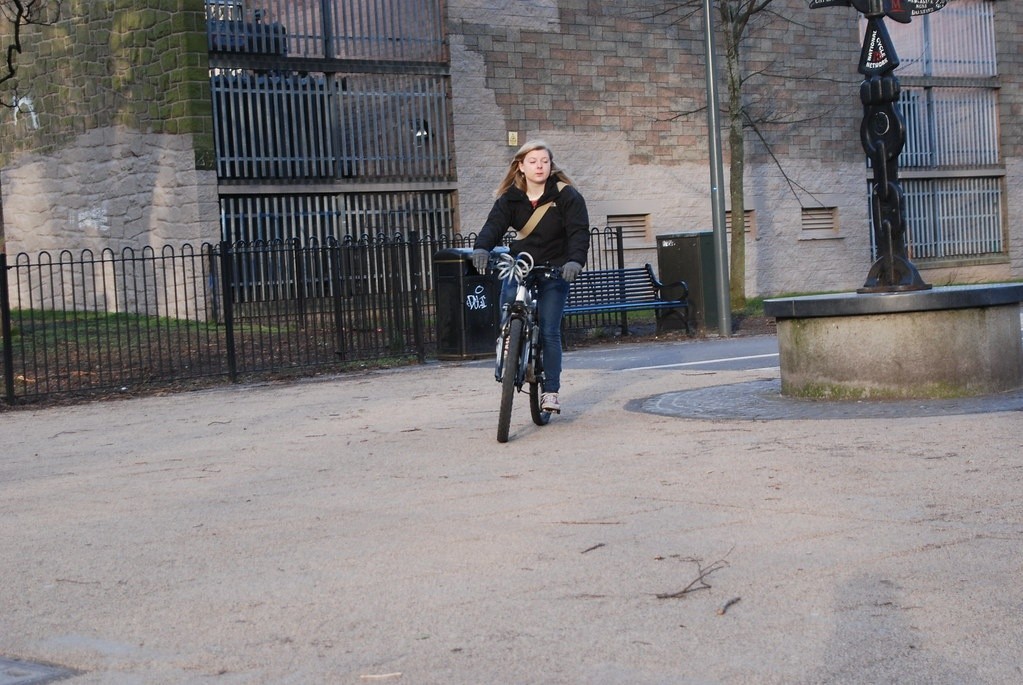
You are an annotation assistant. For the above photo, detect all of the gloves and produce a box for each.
[561,261,582,282]
[472,249,492,268]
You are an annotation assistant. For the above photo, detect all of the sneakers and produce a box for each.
[496,334,509,357]
[540,393,560,411]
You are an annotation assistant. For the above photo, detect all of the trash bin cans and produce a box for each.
[432,247,511,361]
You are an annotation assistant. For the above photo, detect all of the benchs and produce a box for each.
[560,263,693,350]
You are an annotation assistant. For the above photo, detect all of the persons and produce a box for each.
[473,140,590,412]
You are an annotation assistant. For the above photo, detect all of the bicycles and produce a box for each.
[461,253,582,444]
